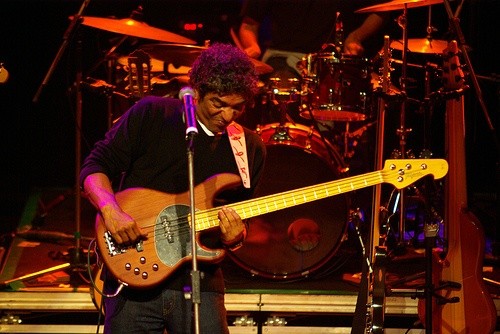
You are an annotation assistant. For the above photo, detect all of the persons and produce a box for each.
[203,0,262,59]
[344,0,433,56]
[82,44,268,334]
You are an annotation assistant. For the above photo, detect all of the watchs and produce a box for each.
[224,235,247,251]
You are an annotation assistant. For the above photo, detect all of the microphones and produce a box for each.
[178,86,199,134]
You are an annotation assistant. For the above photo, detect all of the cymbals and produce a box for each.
[391,38,476,55]
[67,14,200,45]
[353,0,448,13]
[151,40,276,76]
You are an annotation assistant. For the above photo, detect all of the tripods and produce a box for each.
[0,0,105,290]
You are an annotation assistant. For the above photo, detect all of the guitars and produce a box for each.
[94,148,448,292]
[416,39,498,334]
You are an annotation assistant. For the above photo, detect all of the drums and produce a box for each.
[300,51,375,123]
[243,74,308,126]
[217,119,354,282]
